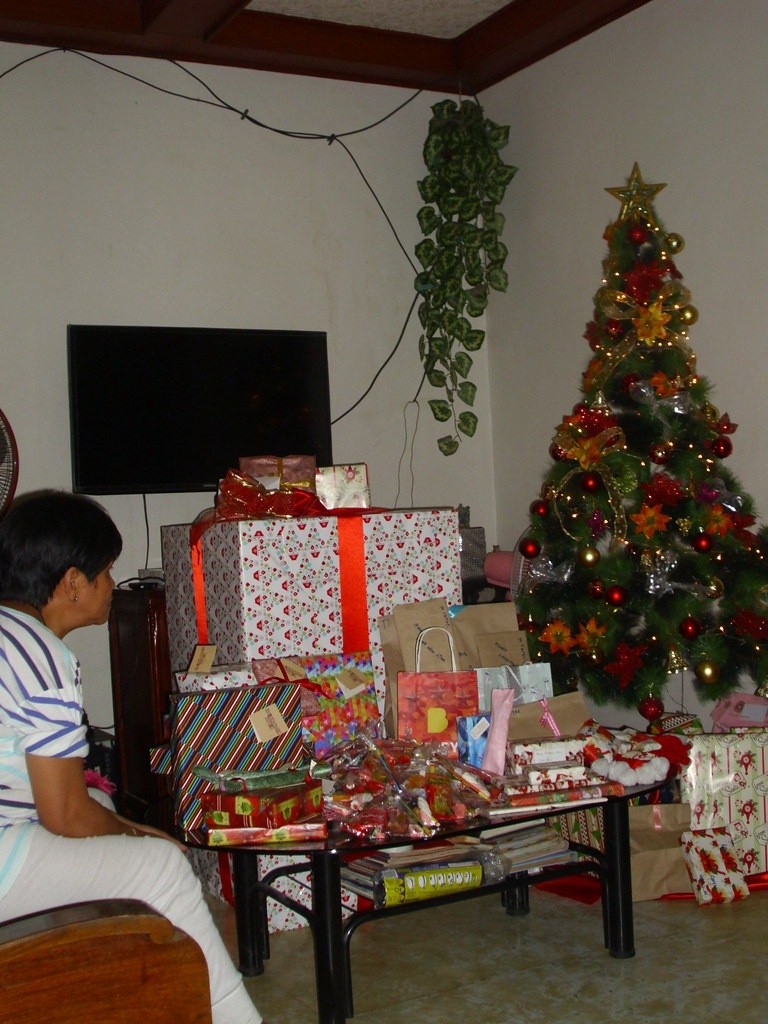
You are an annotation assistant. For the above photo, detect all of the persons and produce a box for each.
[0,488,266,1024]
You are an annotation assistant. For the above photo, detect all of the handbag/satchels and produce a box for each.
[628,804,694,902]
[471,666,510,714]
[512,661,553,708]
[398,627,479,745]
[457,715,491,769]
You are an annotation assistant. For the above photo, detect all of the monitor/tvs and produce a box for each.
[65,325,334,495]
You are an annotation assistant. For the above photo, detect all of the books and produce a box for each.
[339,819,579,901]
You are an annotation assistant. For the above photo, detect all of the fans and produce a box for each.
[0,406,20,522]
[482,523,547,603]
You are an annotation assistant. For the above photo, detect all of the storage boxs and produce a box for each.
[149,454,768,934]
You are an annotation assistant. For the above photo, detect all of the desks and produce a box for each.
[178,774,671,1024]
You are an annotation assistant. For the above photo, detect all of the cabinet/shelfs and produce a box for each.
[106,582,180,834]
